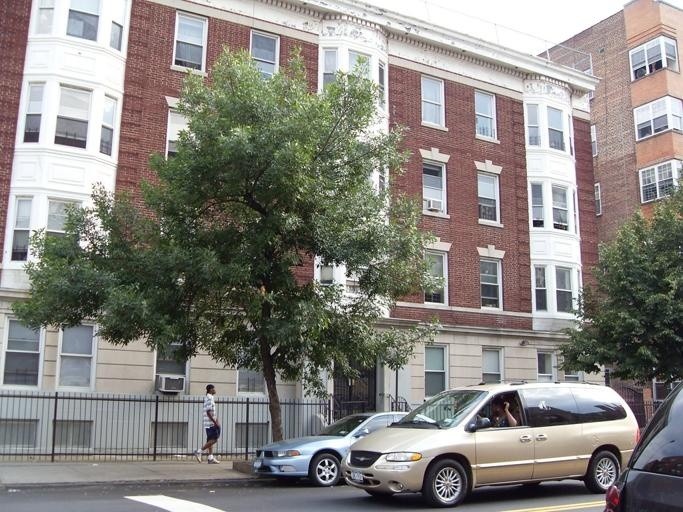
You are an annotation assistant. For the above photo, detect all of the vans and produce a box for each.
[334,377,642,510]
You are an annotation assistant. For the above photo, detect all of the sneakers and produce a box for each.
[208,458,220,464]
[194,450,202,463]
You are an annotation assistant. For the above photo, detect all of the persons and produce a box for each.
[479,398,517,427]
[194,385,221,464]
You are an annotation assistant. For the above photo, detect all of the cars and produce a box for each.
[597,376,682,512]
[251,409,438,490]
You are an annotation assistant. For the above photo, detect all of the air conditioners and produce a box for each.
[157,372,188,395]
